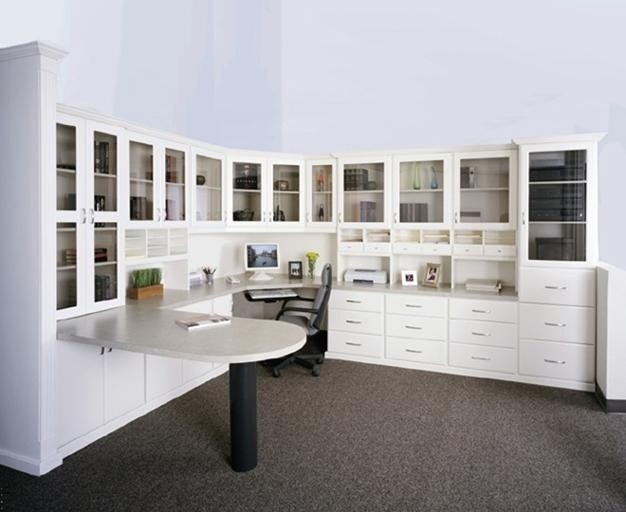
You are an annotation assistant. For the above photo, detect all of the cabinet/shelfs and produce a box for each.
[52,104,607,462]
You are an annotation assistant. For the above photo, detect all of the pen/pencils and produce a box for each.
[201,264,217,274]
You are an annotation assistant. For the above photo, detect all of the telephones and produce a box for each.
[225,274,240,284]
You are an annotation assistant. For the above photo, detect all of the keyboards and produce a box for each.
[250,290,296,298]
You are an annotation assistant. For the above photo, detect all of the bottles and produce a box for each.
[318,169,325,192]
[414,166,420,190]
[432,173,437,189]
[318,204,326,220]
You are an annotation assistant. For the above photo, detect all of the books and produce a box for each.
[400,202,429,224]
[359,200,377,222]
[56,140,114,306]
[174,312,231,333]
[130,155,178,221]
[344,168,370,192]
[462,280,504,294]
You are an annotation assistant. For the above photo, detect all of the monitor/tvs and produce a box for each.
[244,242,278,281]
